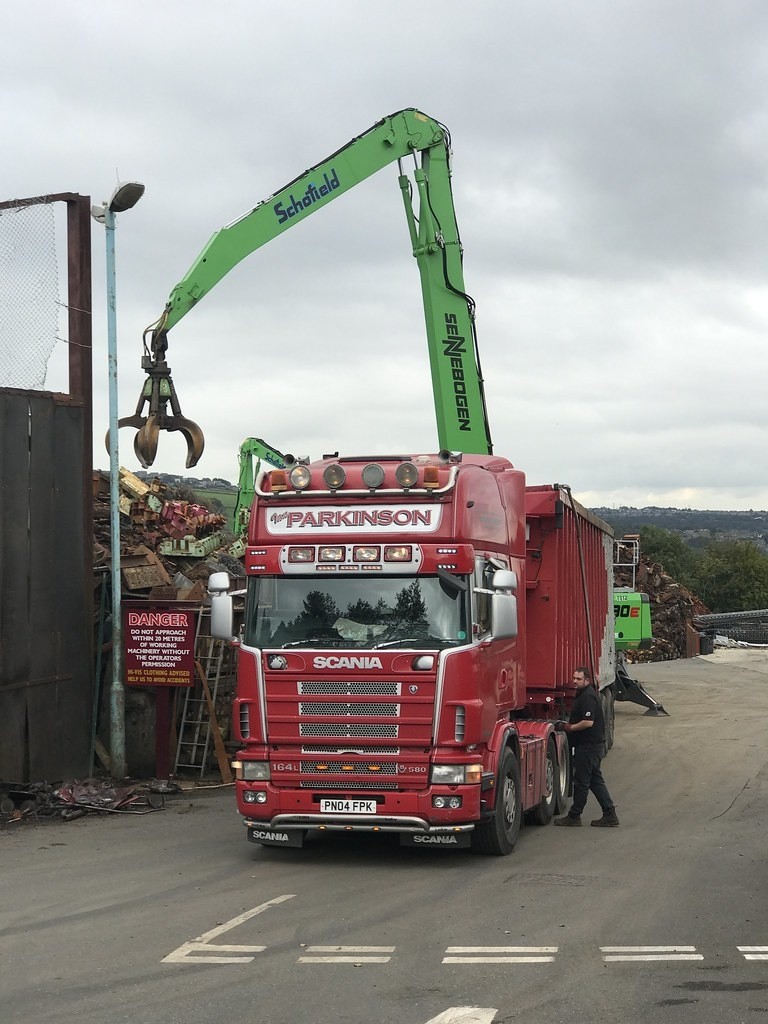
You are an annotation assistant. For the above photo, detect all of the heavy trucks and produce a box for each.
[205,450,619,857]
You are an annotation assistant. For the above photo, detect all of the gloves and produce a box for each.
[554,721,572,731]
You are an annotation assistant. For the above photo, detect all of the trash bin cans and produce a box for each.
[700,636,709,655]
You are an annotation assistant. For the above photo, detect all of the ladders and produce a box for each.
[174,605,225,776]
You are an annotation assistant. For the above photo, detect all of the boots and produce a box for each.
[554,814,581,825]
[591,806,619,826]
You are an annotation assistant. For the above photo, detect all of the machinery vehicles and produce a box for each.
[105,105,674,718]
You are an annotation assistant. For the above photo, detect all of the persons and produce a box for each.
[554,667,619,827]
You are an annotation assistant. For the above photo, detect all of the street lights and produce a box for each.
[102,178,147,784]
